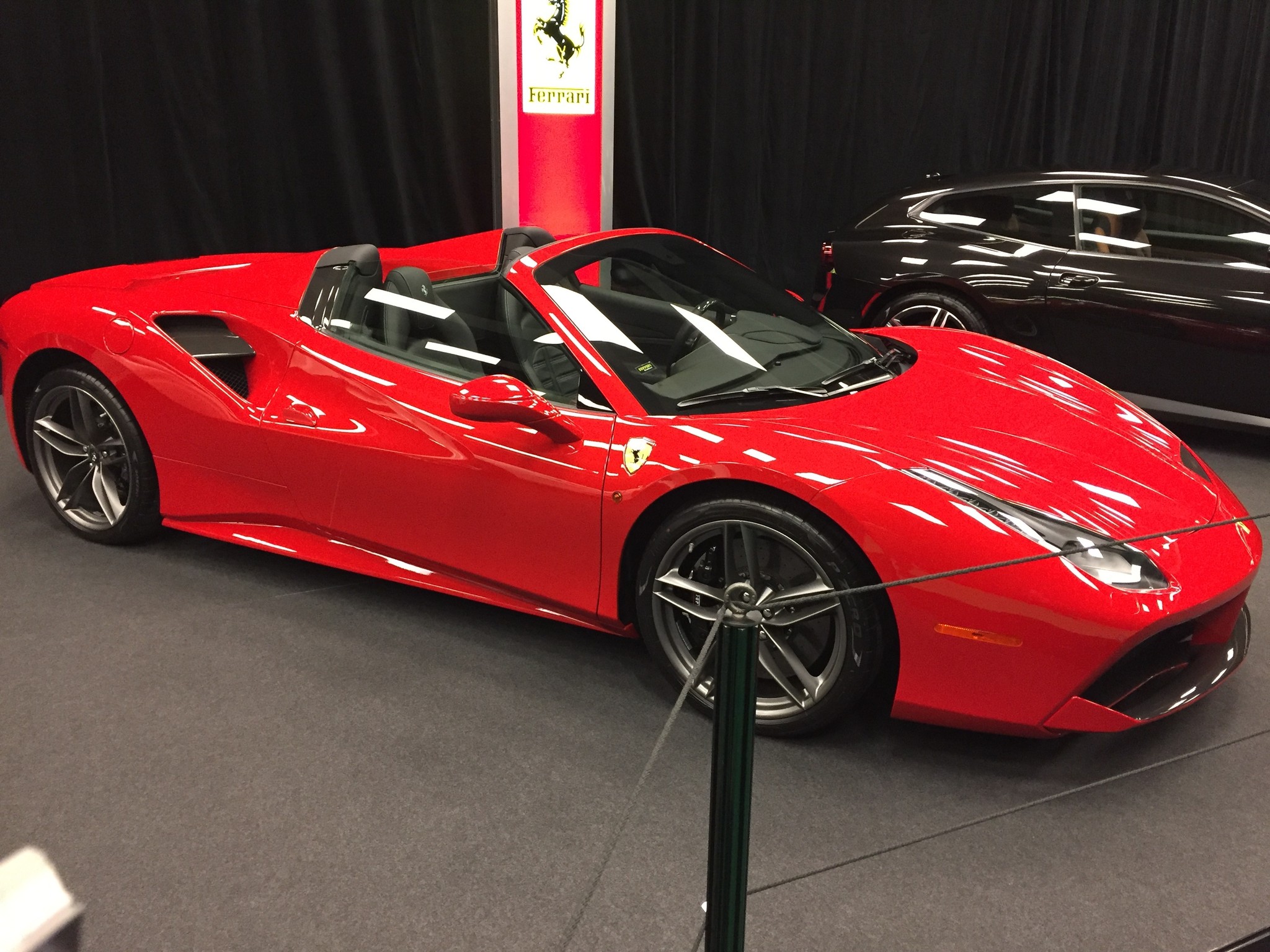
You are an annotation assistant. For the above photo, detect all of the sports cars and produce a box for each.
[0,226,1265,740]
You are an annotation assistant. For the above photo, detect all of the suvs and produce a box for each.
[810,169,1270,438]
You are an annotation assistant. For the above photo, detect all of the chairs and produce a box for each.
[1092,199,1151,257]
[381,266,486,377]
[497,247,584,405]
